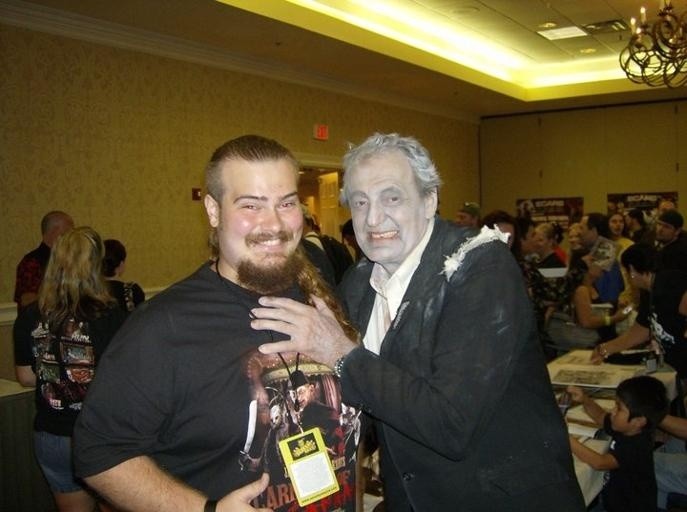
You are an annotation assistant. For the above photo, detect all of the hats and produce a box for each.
[628,209,648,227]
[458,201,481,217]
[658,210,684,228]
[285,370,308,390]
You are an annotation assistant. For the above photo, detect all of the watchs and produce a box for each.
[334,355,345,379]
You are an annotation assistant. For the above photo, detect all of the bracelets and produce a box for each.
[203,499,218,511]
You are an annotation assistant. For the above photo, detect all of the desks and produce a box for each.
[543,349,678,509]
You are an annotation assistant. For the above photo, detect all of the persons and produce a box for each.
[73,133,368,511]
[248,134,587,511]
[458,200,687,511]
[13,211,146,512]
[298,213,365,284]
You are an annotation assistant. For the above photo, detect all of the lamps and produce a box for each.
[618,1,687,89]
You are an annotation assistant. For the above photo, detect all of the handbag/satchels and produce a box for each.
[545,311,617,353]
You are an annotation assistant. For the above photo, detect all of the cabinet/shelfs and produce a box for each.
[0,377,58,511]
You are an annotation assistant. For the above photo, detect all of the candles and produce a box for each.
[640,6,646,24]
[630,17,636,35]
